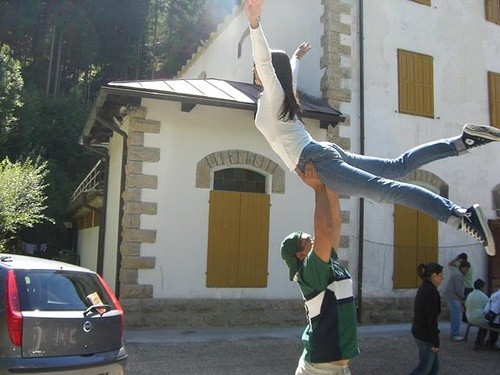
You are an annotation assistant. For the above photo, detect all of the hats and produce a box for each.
[280,231,302,281]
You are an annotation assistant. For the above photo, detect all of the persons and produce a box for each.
[483,289,500,349]
[465,278,489,350]
[445,262,471,341]
[448,253,472,321]
[281,161,360,375]
[244,0,500,256]
[409,262,444,375]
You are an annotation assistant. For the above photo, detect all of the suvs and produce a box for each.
[0,253,128,375]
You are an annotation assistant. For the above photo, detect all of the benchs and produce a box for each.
[464,323,500,353]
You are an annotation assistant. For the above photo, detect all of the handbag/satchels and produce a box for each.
[485,310,496,322]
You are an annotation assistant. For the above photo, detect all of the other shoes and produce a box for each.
[473,345,484,350]
[452,335,463,341]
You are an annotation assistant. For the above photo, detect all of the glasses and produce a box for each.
[302,235,314,251]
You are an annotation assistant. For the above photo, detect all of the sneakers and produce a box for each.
[460,123,500,148]
[458,204,496,256]
